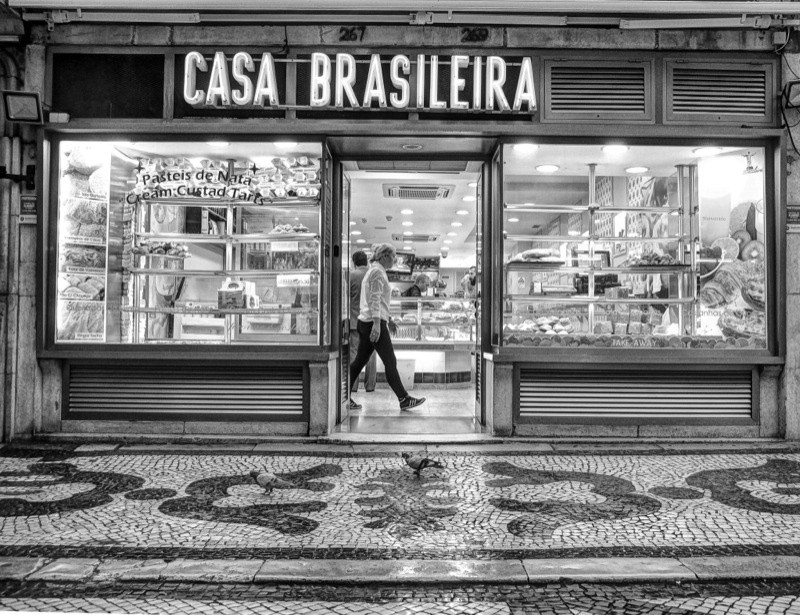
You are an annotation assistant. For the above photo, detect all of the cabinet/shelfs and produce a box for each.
[139,195,324,345]
[376,297,476,383]
[508,162,697,335]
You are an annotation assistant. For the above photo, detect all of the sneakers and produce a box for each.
[350,398,362,409]
[399,396,425,410]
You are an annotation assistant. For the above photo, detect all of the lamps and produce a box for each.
[2,89,45,126]
[786,78,800,108]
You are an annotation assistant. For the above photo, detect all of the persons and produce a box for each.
[350,244,425,410]
[350,252,376,392]
